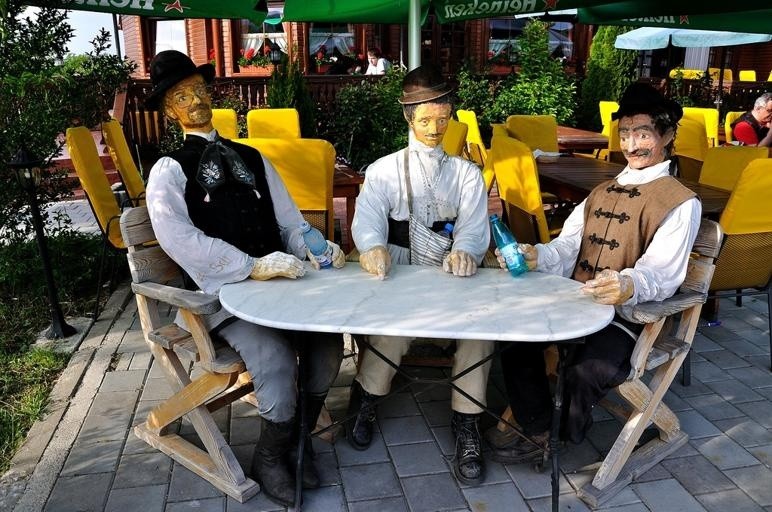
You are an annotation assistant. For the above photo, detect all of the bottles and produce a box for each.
[435,223,454,238]
[299,221,333,268]
[490,214,528,276]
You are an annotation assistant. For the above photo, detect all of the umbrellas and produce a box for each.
[614,26,772,50]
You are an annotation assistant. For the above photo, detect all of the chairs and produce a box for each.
[669,67,772,100]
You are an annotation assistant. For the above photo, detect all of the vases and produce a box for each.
[492,64,511,74]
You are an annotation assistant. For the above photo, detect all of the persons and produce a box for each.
[729,92,772,147]
[491,81,703,465]
[345,65,491,485]
[142,50,345,508]
[364,47,391,76]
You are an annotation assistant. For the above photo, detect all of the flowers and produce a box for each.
[207,44,367,76]
[487,50,510,63]
